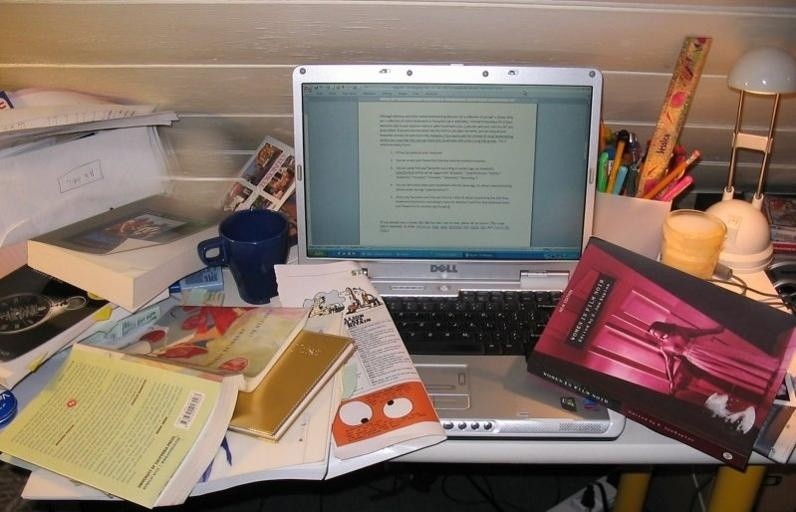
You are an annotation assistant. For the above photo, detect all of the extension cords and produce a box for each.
[547,476,618,512]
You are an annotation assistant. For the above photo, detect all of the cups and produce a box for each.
[595,190,675,266]
[197,209,290,304]
[660,209,728,280]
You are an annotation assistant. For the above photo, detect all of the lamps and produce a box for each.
[703,44,796,276]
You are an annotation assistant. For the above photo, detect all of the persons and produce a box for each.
[647,317,781,400]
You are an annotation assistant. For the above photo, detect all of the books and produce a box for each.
[1,137,451,509]
[525,234,796,474]
[752,372,796,466]
[740,190,796,244]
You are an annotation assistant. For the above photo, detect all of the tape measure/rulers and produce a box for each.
[634,37,713,198]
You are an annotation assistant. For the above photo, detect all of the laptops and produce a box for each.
[292,64,626,439]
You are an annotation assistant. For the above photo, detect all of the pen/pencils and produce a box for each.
[596,117,701,201]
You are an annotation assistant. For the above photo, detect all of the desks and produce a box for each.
[0,271,795,511]
